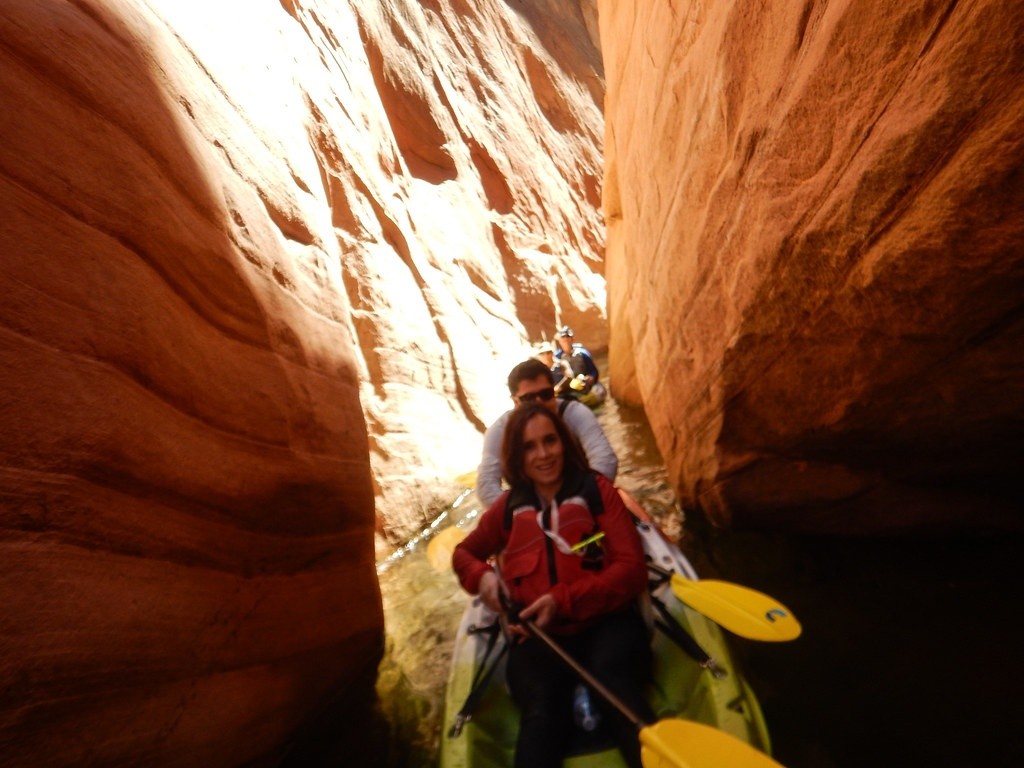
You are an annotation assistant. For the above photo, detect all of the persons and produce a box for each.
[475,359,620,509]
[537,341,574,394]
[450,400,654,768]
[555,327,599,384]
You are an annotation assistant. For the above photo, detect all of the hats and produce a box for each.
[538,341,553,354]
[559,326,574,337]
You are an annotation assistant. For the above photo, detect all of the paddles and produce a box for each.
[499,599,779,768]
[644,562,801,642]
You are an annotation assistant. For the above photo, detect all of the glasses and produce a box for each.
[511,387,554,404]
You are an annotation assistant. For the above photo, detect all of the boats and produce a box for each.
[440,488,771,768]
[563,380,607,410]
